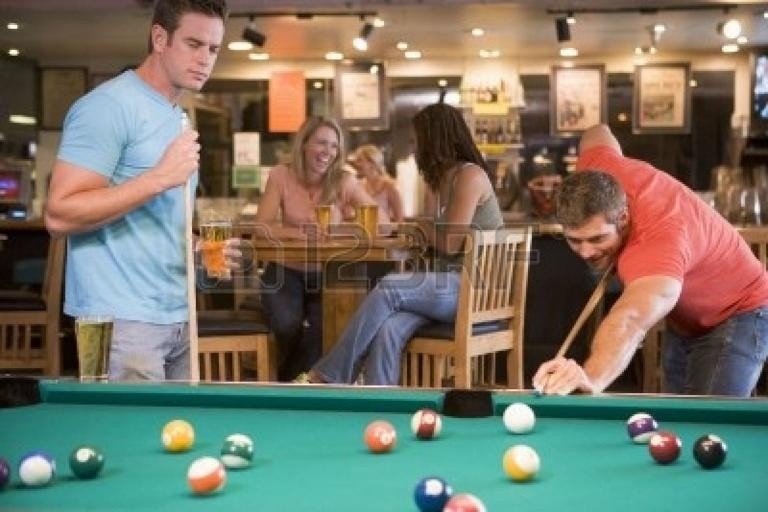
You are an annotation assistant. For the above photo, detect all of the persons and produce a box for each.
[499,112,565,223]
[530,124,768,398]
[290,104,504,383]
[353,145,401,275]
[258,115,390,373]
[44,1,231,384]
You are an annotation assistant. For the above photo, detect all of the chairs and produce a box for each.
[193,309,278,380]
[399,227,532,388]
[635,226,768,394]
[0,234,68,380]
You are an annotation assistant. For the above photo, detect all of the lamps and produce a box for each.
[239,15,266,46]
[716,7,742,40]
[354,17,374,51]
[553,10,575,45]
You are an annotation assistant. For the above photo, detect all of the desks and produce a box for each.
[1,373,768,512]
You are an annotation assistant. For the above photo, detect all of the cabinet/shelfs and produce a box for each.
[457,104,527,210]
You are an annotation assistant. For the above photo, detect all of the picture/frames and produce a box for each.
[547,62,609,139]
[332,58,388,132]
[37,65,89,130]
[631,60,694,135]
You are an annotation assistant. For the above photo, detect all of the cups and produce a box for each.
[199,224,232,279]
[354,203,377,238]
[313,204,332,239]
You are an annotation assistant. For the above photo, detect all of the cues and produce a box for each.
[534,260,619,396]
[181,111,201,384]
